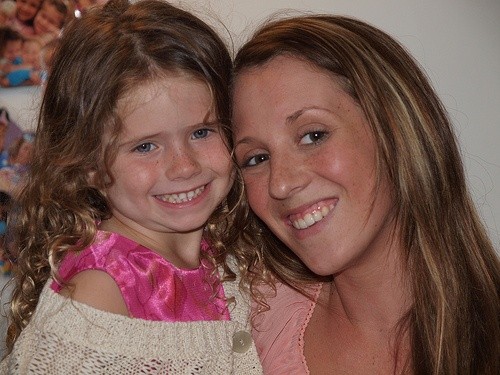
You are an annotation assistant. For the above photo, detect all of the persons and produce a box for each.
[0,0,277,375]
[0,0,69,87]
[229,14,500,375]
